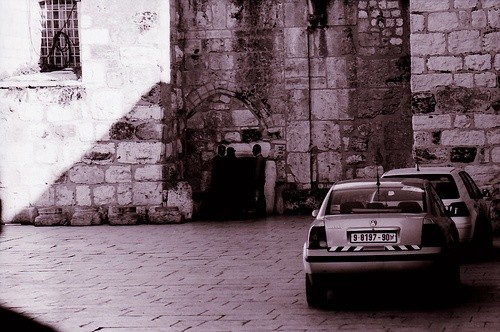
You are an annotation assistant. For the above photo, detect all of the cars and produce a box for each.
[369,166,492,263]
[303,179,460,310]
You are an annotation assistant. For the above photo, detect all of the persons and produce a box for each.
[211,145,227,221]
[249,143,266,218]
[224,147,241,219]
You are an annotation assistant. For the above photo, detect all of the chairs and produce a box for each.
[398,200,422,213]
[340,201,364,214]
